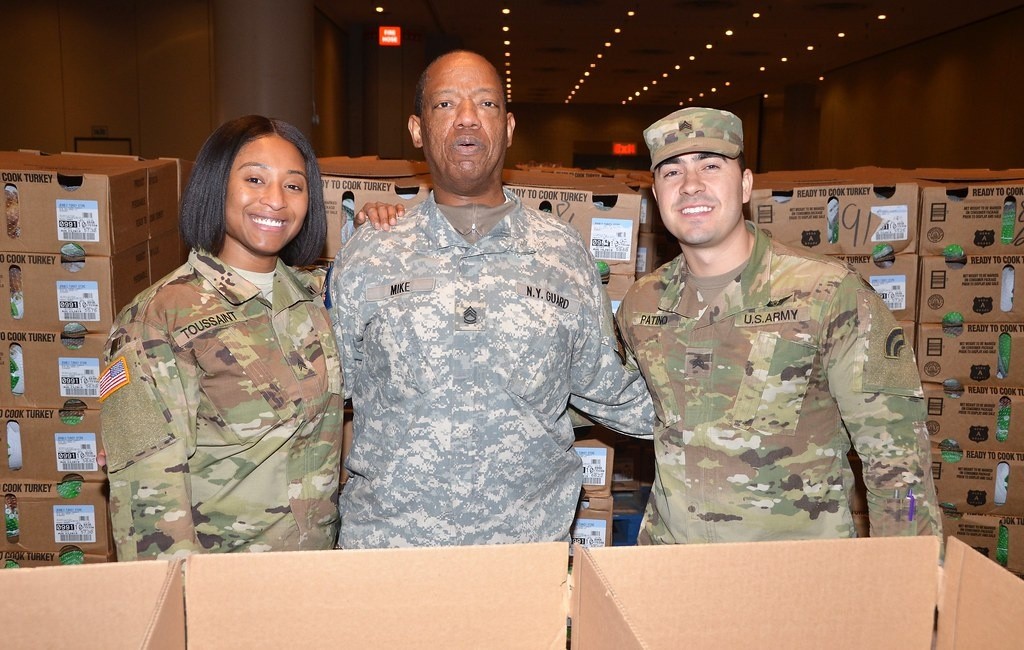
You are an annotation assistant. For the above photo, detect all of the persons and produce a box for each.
[566,107,944,606]
[98,115,404,650]
[95,50,657,557]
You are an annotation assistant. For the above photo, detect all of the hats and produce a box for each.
[643,107,744,172]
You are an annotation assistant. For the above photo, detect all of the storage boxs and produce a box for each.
[0,148,1024,650]
